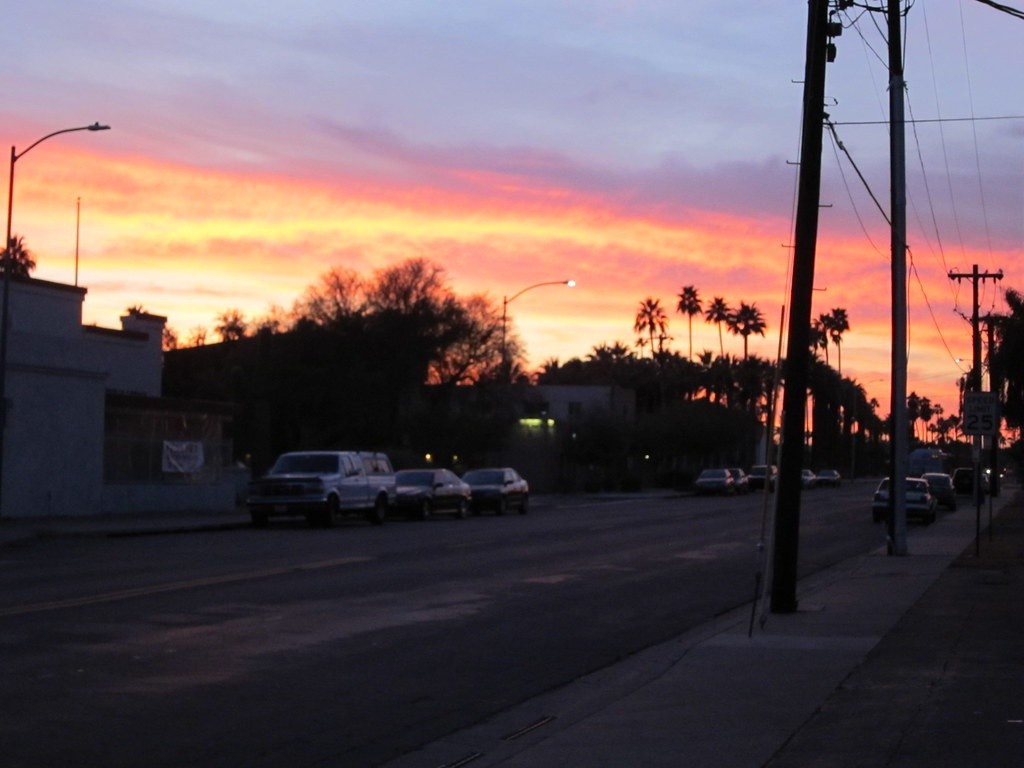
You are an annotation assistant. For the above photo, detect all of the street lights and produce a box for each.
[0,122,112,477]
[500,279,579,470]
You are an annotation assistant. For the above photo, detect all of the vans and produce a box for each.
[246,450,397,529]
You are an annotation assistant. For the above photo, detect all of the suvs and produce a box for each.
[747,465,778,493]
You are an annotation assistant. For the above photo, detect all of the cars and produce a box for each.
[692,468,737,499]
[799,469,818,491]
[461,468,531,519]
[920,472,958,512]
[953,465,1007,496]
[393,469,472,522]
[728,468,750,494]
[816,469,844,490]
[870,476,938,527]
